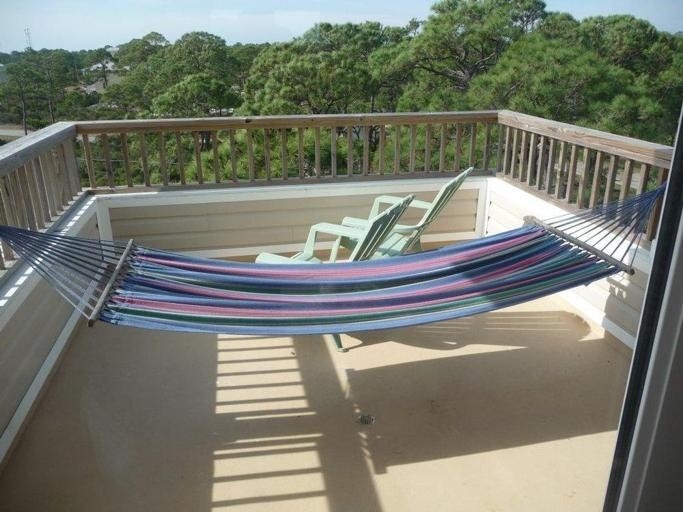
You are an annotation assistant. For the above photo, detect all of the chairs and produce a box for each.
[333,164,476,261]
[251,193,417,354]
